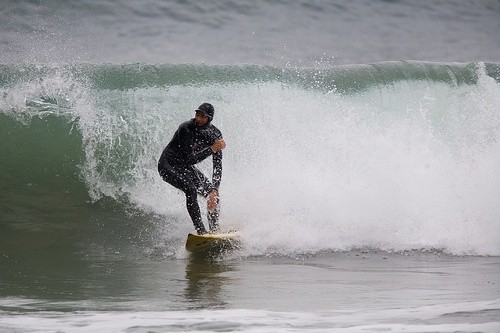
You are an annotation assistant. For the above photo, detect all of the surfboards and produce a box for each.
[185,230,243,251]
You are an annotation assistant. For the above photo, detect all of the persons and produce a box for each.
[157,101,225,235]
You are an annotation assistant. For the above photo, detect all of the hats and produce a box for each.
[195,102,214,117]
